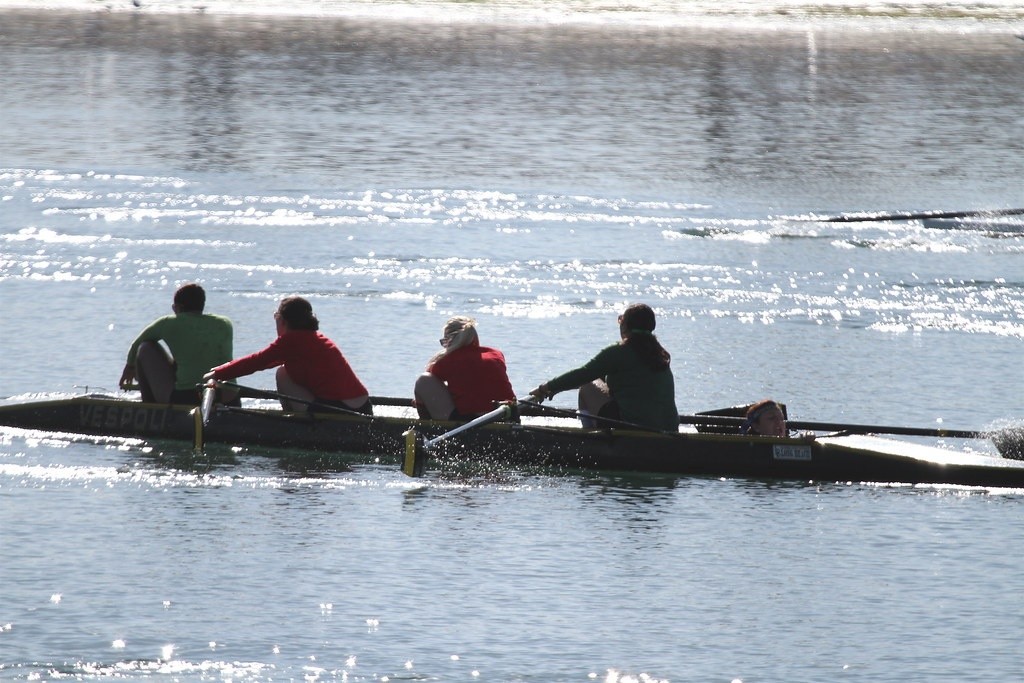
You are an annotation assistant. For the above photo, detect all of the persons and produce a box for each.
[529,304,679,432]
[415,317,520,423]
[202,296,372,415]
[747,401,786,437]
[120,284,241,407]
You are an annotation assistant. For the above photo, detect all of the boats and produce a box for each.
[0,390,1024,491]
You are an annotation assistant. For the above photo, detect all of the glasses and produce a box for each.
[440,338,448,347]
[618,315,624,325]
[274,312,285,323]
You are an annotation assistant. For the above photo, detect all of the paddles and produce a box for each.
[676,412,1024,460]
[517,402,786,432]
[236,383,416,406]
[401,392,540,477]
[187,379,216,453]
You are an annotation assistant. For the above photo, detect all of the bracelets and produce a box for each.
[126,364,134,369]
[539,384,546,393]
